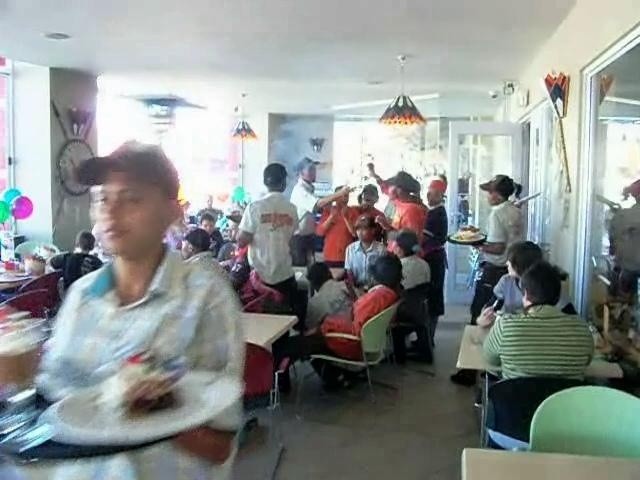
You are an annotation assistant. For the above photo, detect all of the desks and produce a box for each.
[456,324,624,447]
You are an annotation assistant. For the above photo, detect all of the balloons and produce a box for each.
[1,186,22,204]
[0,201,10,224]
[10,196,34,220]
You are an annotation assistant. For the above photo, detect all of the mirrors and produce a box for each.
[573,20,640,398]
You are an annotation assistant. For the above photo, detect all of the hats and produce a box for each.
[296,158,318,172]
[356,214,376,229]
[386,171,420,192]
[264,162,287,185]
[76,140,180,196]
[481,175,514,196]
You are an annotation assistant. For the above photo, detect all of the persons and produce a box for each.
[29,135,248,479]
[459,170,597,406]
[608,180,640,298]
[46,154,448,406]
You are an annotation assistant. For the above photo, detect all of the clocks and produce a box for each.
[57,139,95,197]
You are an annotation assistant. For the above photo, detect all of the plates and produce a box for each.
[450,234,486,242]
[40,370,246,447]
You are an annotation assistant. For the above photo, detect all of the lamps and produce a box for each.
[232,93,258,141]
[377,55,427,129]
[543,72,570,118]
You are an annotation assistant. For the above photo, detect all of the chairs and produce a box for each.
[530,385,640,456]
[488,375,597,450]
